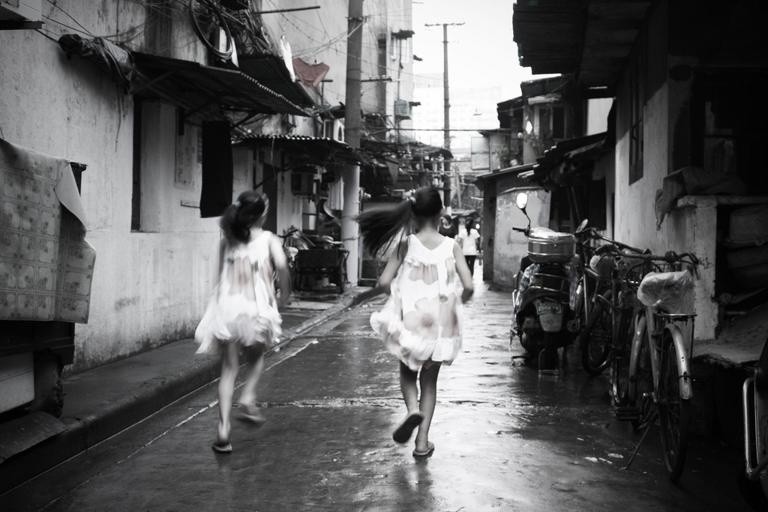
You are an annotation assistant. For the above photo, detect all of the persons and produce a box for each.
[456,219,481,278]
[192,191,298,454]
[354,184,474,457]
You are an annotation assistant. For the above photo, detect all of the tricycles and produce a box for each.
[294,231,354,294]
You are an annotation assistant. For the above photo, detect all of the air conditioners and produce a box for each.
[0,0,43,24]
[291,172,317,197]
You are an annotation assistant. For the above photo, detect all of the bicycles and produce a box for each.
[574,224,700,485]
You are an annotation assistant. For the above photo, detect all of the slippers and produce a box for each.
[414,441,434,455]
[211,412,265,452]
[394,412,423,442]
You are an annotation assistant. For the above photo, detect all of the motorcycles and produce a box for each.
[508,191,587,377]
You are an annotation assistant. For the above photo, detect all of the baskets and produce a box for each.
[626,263,676,293]
